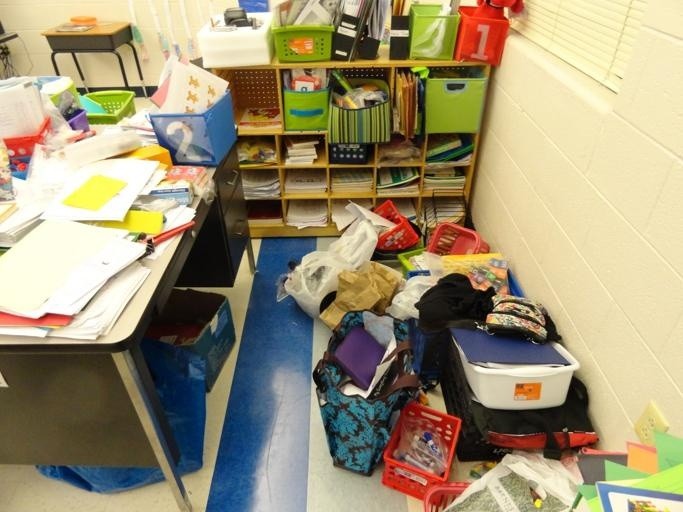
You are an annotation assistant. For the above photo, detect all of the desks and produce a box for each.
[0,100,256,512]
[41,22,147,101]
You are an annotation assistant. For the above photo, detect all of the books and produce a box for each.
[233,134,476,197]
[229,196,475,231]
[237,106,282,129]
[52,22,96,32]
[131,157,213,218]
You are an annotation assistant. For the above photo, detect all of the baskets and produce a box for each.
[3,116,50,170]
[375,200,527,511]
[271,18,335,62]
[328,142,368,165]
[83,91,136,125]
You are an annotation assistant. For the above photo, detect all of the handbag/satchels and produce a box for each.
[471,378,598,449]
[315,309,419,476]
[486,294,549,342]
[36,340,207,493]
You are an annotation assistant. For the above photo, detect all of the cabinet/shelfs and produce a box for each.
[212,49,494,239]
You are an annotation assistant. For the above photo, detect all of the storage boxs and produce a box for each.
[149,90,237,165]
[141,288,238,392]
[453,338,580,413]
[329,78,393,144]
[410,4,460,61]
[455,5,509,67]
[426,67,488,135]
[284,88,328,131]
